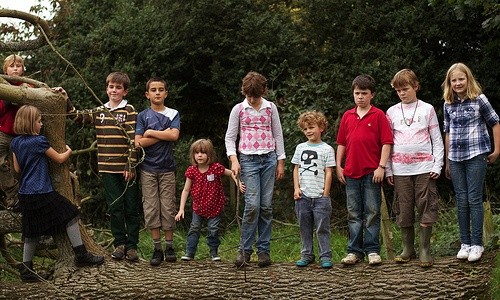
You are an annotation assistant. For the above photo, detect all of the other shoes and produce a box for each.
[320,256,333,268]
[296,257,311,266]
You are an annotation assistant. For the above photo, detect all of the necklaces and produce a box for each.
[401,99,418,126]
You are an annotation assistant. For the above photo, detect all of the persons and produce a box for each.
[52,72,139,262]
[10,105,104,282]
[336,75,394,265]
[0,55,36,213]
[385,68,444,266]
[175,139,246,261]
[291,110,336,268]
[225,72,286,267]
[135,78,180,266]
[440,63,500,261]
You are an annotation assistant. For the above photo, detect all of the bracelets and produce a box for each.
[379,164,385,169]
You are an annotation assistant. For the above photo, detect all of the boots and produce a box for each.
[395,227,416,262]
[17,261,48,281]
[72,244,104,267]
[418,226,433,267]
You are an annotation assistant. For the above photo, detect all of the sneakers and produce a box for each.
[164,247,177,262]
[468,244,484,261]
[367,252,382,265]
[457,243,471,259]
[258,252,271,266]
[150,248,164,265]
[235,254,250,265]
[181,254,194,261]
[210,253,221,261]
[126,248,139,261]
[341,253,364,264]
[111,244,126,259]
